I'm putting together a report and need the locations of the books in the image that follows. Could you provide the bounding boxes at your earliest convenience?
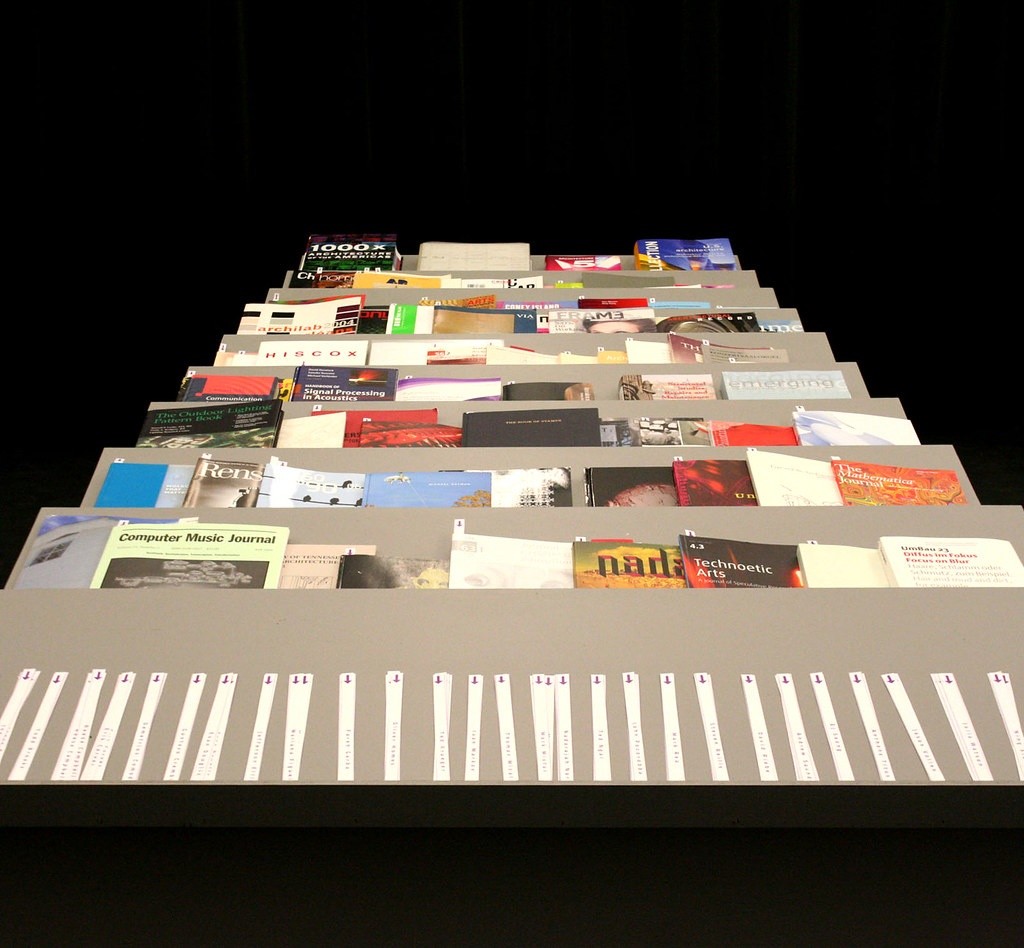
[16,231,968,593]
[879,535,1024,589]
